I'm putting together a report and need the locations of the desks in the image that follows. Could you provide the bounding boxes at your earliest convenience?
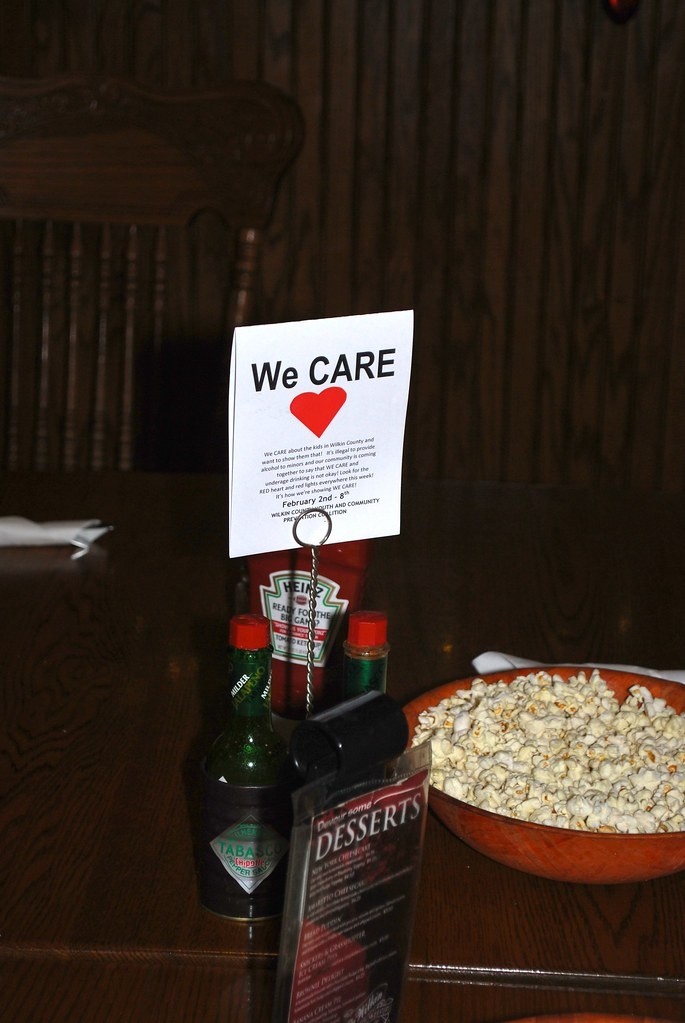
[0,471,685,1023]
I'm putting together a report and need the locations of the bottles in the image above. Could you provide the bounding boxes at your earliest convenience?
[246,534,367,719]
[340,612,390,697]
[187,614,291,920]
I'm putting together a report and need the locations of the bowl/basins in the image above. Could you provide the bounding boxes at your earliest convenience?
[398,666,684,887]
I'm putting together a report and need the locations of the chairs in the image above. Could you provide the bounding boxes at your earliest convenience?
[1,72,304,474]
